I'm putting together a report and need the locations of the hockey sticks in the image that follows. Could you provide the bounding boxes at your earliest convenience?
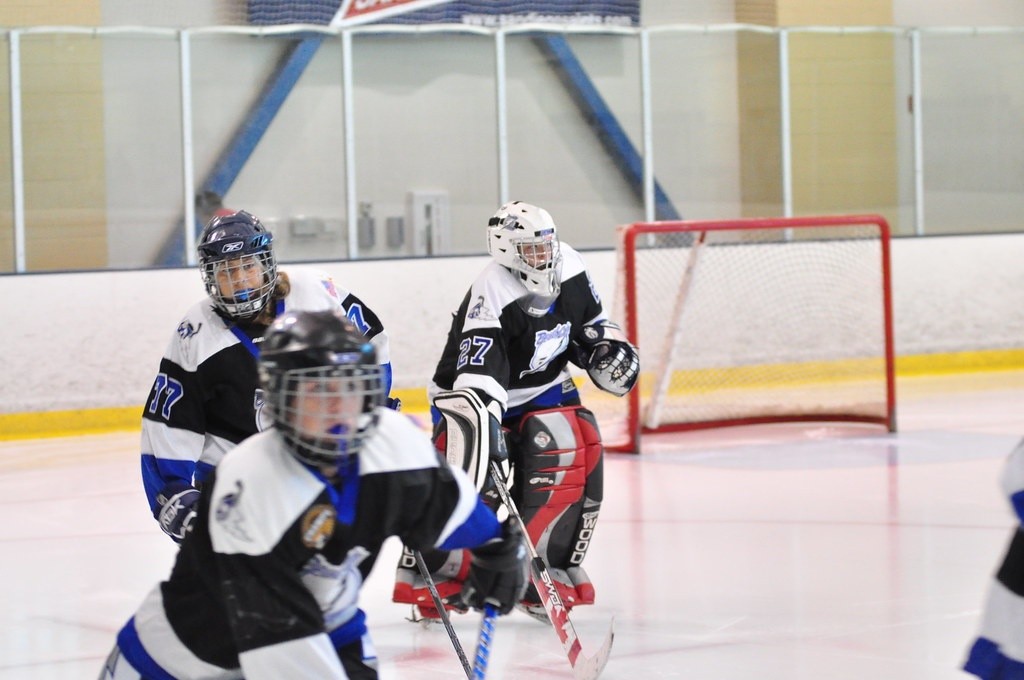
[469,598,501,680]
[488,460,617,680]
[412,550,472,678]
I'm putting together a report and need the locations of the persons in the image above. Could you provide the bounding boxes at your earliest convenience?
[96,312,500,680]
[960,435,1024,680]
[140,210,389,544]
[195,191,236,226]
[392,202,639,622]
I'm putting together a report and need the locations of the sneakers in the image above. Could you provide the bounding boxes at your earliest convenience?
[514,602,552,626]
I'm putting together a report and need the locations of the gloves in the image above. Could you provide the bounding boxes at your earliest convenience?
[159,487,201,545]
[460,523,529,615]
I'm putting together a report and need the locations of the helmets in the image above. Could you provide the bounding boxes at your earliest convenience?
[198,209,272,320]
[487,200,556,289]
[261,309,376,463]
